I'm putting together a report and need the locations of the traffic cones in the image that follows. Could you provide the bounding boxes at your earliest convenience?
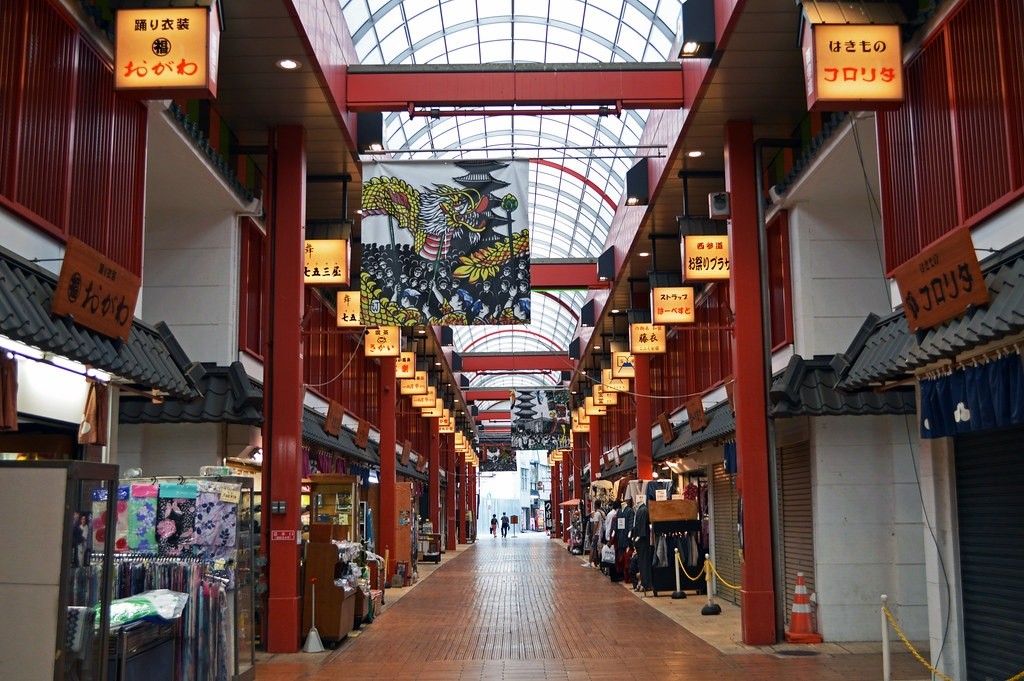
[784,572,823,644]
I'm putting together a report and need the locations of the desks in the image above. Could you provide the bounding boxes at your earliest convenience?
[93,610,184,681]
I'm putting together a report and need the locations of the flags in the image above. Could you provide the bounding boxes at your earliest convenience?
[357,157,532,326]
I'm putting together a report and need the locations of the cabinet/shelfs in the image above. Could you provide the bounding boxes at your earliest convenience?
[418,533,441,564]
[307,474,361,541]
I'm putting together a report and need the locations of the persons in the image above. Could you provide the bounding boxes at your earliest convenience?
[581,499,617,570]
[491,514,499,538]
[612,502,652,593]
[500,512,509,538]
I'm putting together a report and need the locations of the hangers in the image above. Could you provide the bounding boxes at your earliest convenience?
[215,474,222,482]
[179,475,184,484]
[91,552,204,563]
[151,476,157,484]
